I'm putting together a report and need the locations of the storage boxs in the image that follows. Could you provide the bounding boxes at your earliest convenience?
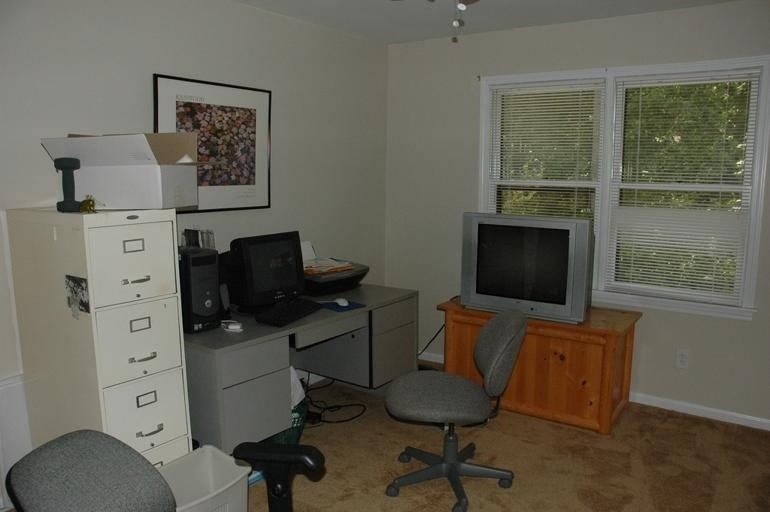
[38,132,198,210]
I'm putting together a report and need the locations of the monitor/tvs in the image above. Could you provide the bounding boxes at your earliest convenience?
[219,231,307,313]
[462,212,595,325]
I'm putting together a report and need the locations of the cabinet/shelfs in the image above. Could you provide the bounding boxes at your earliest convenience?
[436,297,642,433]
[7,208,193,469]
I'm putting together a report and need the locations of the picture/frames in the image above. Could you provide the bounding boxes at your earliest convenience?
[154,74,271,212]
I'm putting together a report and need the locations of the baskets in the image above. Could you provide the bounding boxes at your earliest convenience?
[271,410,306,445]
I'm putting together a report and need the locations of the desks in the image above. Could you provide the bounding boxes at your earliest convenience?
[184,284,419,455]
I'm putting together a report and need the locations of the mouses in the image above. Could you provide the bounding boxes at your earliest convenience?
[334,297,350,307]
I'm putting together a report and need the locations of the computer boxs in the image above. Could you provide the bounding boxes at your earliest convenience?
[178,248,220,319]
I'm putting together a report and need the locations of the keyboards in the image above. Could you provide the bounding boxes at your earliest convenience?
[255,298,323,327]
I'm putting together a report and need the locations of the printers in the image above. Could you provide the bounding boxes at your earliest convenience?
[300,240,371,297]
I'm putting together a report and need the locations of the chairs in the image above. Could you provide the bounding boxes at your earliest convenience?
[5,429,177,512]
[383,310,527,512]
